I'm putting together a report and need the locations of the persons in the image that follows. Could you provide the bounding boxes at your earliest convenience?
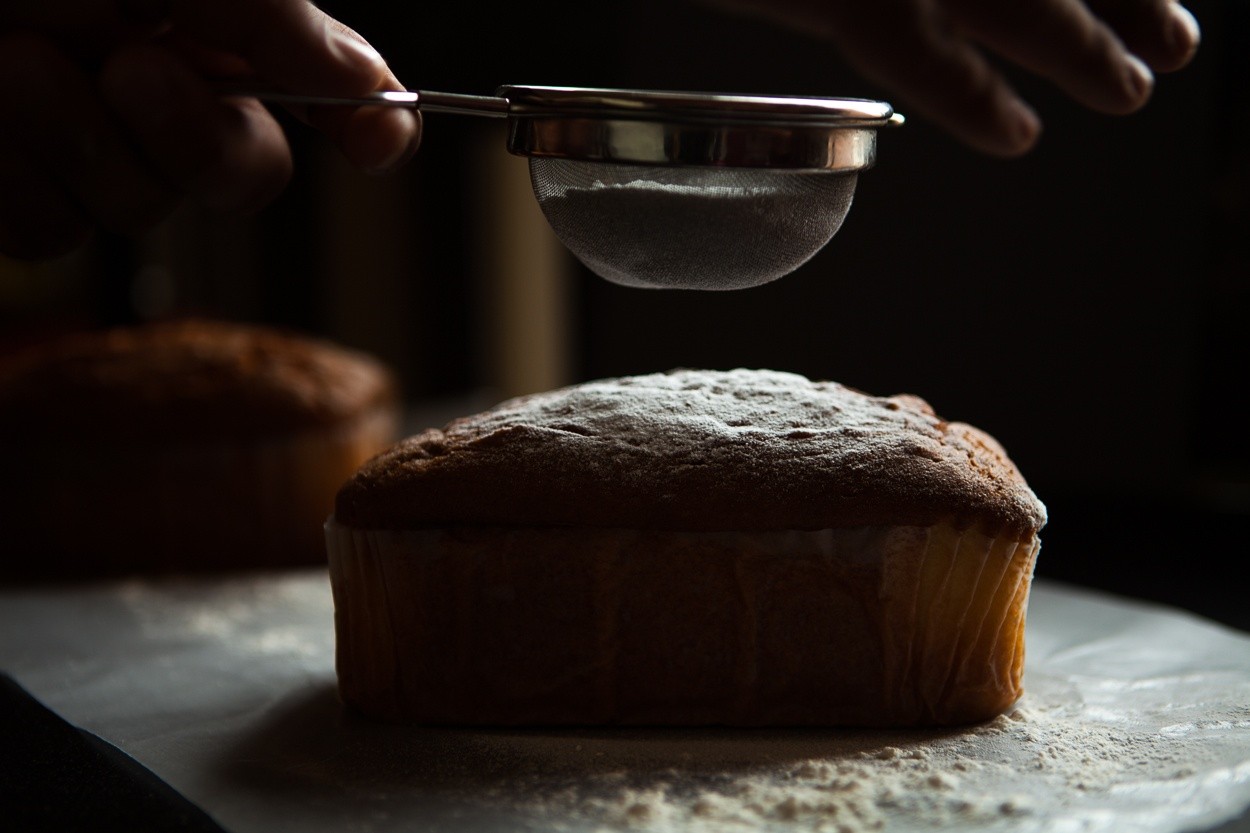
[0,0,1250,631]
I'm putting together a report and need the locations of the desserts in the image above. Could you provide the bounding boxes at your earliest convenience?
[0,325,398,578]
[323,368,1048,731]
